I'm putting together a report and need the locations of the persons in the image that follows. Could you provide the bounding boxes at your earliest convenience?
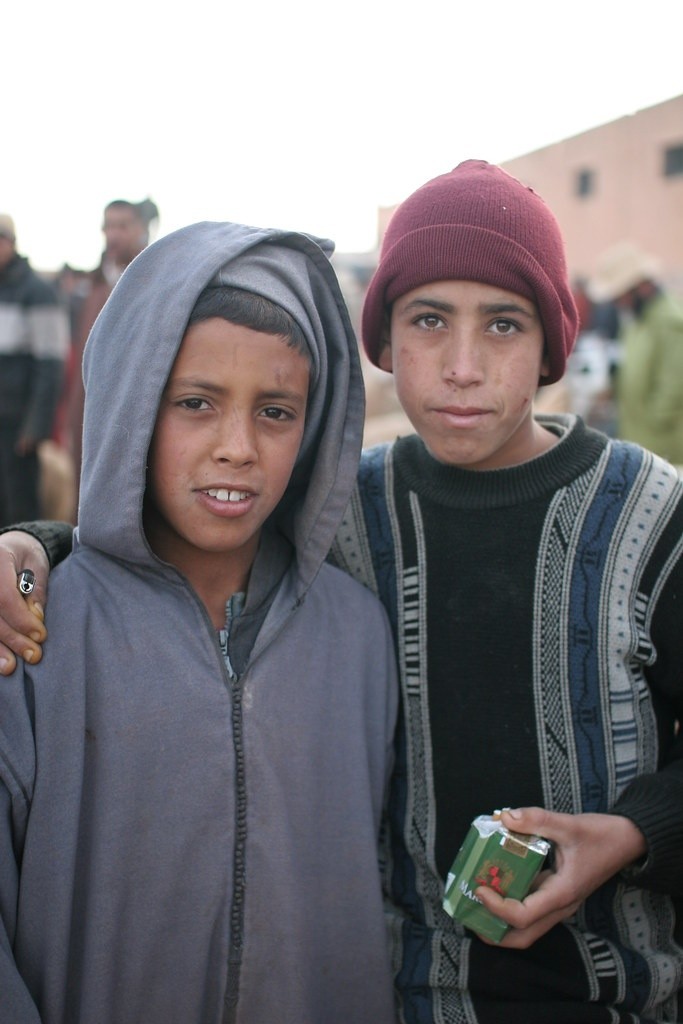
[0,222,404,1024]
[555,243,683,467]
[0,199,158,532]
[0,157,683,1024]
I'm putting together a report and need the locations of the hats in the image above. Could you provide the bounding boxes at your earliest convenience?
[361,158,580,387]
[584,240,661,303]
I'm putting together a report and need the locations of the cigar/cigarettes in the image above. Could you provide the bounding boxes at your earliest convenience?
[492,809,502,820]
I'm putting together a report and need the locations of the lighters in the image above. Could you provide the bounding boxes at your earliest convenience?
[16,568,35,598]
